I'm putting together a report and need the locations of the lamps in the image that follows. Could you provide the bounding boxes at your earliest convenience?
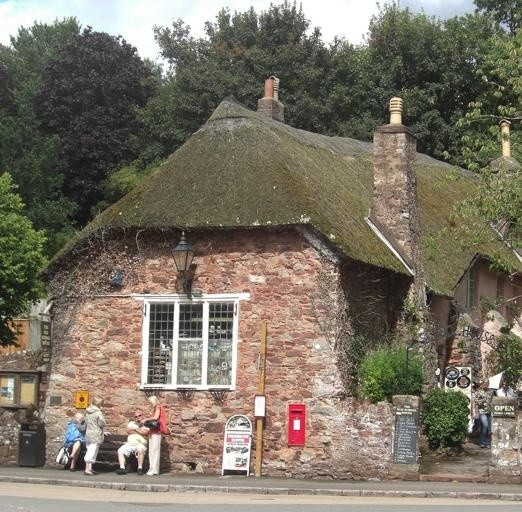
[168,232,195,286]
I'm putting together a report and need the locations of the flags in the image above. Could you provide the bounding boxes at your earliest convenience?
[488,372,502,390]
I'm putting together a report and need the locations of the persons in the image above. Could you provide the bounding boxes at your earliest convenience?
[465,377,520,450]
[115,396,167,476]
[55,396,106,476]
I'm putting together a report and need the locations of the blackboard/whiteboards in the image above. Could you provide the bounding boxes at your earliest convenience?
[392,394,420,465]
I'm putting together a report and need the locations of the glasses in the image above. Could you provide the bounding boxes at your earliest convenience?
[134,414,141,418]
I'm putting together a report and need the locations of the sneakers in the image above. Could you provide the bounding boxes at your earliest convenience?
[145,470,158,476]
[115,467,144,475]
[70,467,81,472]
[84,469,100,476]
[67,455,74,461]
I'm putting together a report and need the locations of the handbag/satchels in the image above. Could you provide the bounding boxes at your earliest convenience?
[143,418,161,428]
[54,445,72,466]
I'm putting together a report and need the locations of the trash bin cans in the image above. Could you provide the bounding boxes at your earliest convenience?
[19,417,46,467]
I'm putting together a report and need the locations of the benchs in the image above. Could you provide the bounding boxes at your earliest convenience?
[63,432,149,474]
